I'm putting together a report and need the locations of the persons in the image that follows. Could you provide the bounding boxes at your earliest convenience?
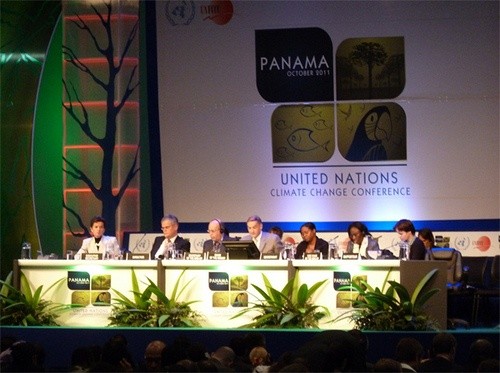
[239,215,285,259]
[150,215,191,260]
[346,222,380,259]
[393,219,425,260]
[0,329,500,373]
[203,218,239,260]
[418,229,441,251]
[73,217,123,260]
[294,223,329,259]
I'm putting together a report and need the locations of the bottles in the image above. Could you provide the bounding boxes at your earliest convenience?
[21,240,31,259]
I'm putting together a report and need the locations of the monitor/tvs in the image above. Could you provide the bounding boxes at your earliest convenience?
[222,240,260,259]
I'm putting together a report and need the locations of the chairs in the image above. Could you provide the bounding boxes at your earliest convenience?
[475,256,500,327]
[460,257,489,327]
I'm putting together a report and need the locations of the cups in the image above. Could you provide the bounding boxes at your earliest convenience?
[330,246,335,259]
[401,243,407,260]
[286,244,292,259]
[66,250,73,259]
[177,250,183,259]
[118,251,123,260]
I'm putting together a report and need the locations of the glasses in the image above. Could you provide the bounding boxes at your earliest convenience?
[160,227,170,230]
[207,229,215,233]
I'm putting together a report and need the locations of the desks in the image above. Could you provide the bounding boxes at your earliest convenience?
[13,258,447,330]
